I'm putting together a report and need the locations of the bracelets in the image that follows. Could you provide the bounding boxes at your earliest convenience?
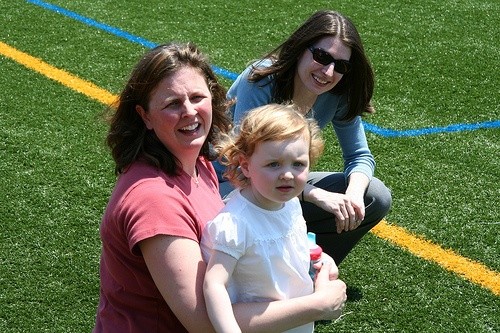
[302,190,305,202]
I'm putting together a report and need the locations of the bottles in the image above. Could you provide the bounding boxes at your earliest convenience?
[307,232,322,284]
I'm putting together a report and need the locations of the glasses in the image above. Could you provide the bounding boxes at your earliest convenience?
[308,45,352,75]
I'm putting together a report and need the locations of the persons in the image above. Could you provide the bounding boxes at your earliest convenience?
[207,11,391,299]
[96,43,348,333]
[199,103,316,333]
